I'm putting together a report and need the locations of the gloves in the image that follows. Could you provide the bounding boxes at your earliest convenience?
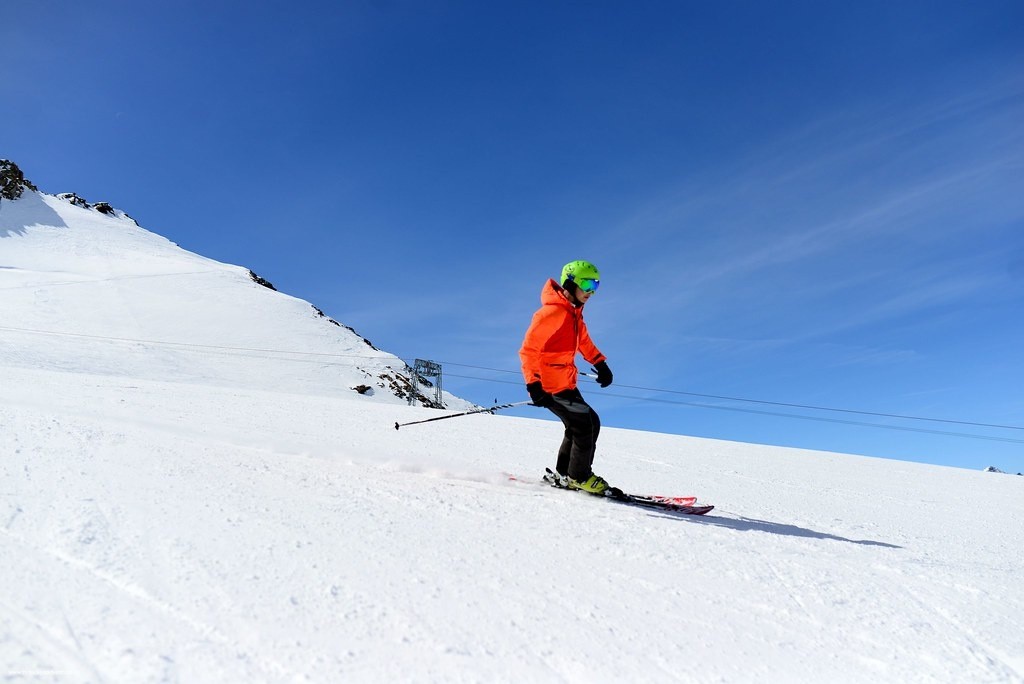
[594,361,613,388]
[526,380,552,408]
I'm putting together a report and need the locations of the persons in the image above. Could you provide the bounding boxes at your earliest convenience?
[519,261,613,493]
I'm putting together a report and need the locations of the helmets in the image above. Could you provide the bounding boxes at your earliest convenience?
[560,260,600,287]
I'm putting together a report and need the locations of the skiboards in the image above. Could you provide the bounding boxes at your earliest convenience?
[543,463,715,516]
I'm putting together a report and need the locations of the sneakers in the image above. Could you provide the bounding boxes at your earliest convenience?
[555,471,568,487]
[567,473,608,494]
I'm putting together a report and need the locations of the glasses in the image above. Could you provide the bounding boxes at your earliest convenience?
[580,279,600,292]
